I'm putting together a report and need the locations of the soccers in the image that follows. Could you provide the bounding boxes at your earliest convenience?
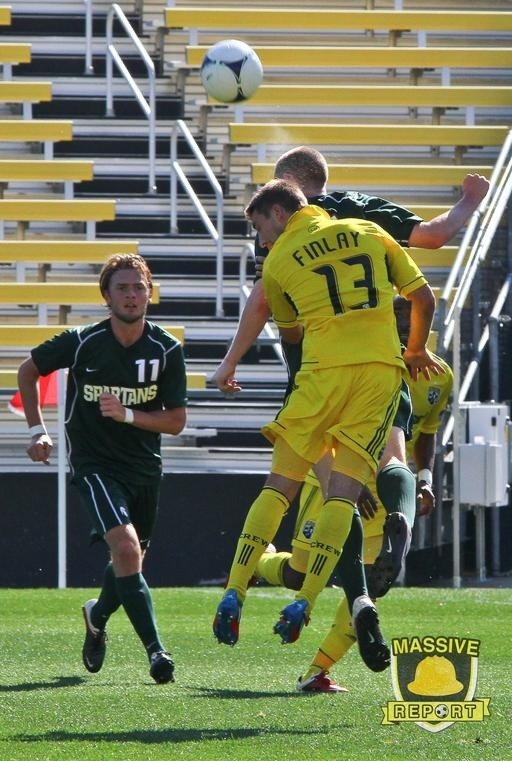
[197,38,264,105]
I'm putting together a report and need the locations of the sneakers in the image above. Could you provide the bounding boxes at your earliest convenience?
[81,597,109,674]
[210,585,244,647]
[147,650,175,686]
[223,542,277,591]
[272,599,309,646]
[297,668,348,697]
[350,596,392,675]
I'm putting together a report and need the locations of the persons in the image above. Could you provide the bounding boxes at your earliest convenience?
[210,178,444,648]
[295,292,456,697]
[19,252,188,687]
[249,146,492,678]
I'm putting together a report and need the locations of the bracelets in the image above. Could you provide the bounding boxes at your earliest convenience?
[124,407,135,425]
[28,424,48,438]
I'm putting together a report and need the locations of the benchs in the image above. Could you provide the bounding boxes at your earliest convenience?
[1,0,512,476]
